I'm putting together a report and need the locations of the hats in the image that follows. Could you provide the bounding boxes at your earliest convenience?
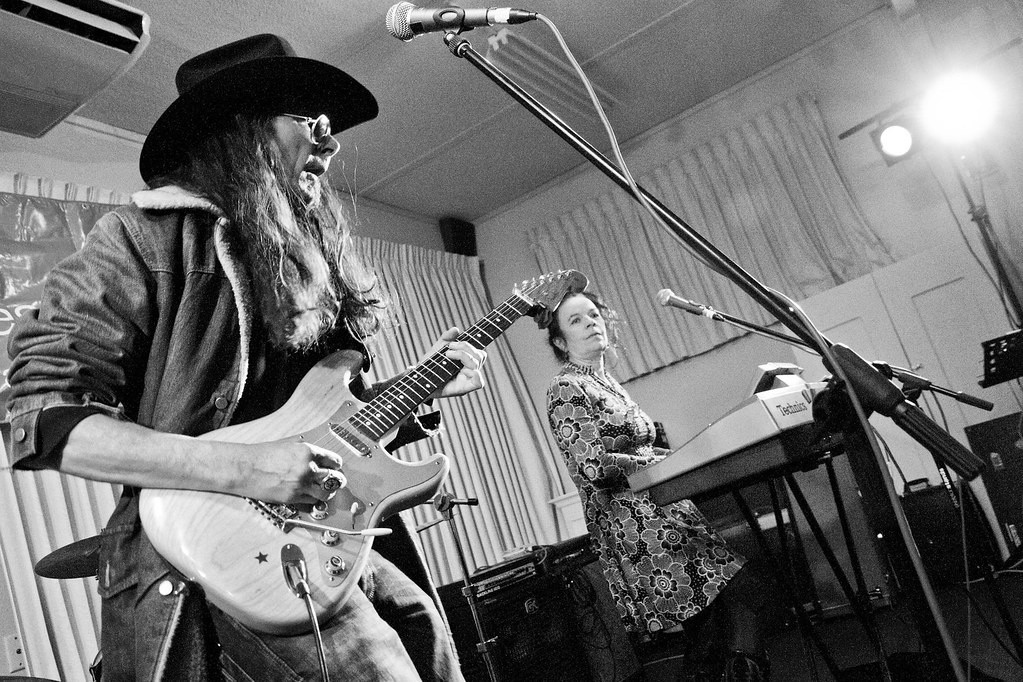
[138,32,380,190]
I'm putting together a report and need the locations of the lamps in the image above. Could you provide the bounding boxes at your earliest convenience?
[486,28,623,127]
[837,30,1022,329]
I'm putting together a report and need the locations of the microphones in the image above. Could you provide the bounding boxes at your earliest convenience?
[656,288,724,322]
[386,0,538,42]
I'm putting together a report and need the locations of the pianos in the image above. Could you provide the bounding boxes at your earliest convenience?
[624,360,862,507]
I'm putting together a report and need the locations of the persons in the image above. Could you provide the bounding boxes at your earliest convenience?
[544,290,751,682]
[0,31,488,682]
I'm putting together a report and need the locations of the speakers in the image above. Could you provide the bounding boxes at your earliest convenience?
[436,532,641,682]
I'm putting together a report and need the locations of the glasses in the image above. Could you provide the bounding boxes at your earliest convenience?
[280,112,332,145]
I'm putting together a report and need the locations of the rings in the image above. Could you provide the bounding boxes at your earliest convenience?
[318,468,342,492]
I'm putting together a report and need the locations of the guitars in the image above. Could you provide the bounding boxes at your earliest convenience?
[137,269,588,635]
